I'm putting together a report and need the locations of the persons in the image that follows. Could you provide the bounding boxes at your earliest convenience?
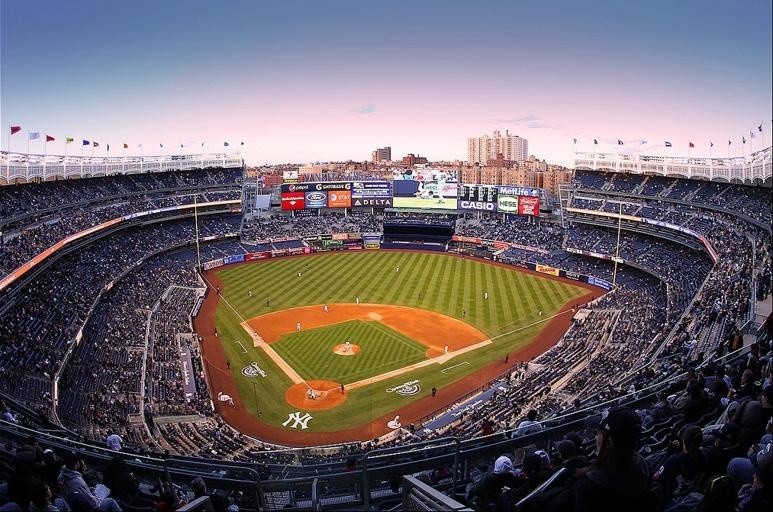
[339,383,346,394]
[307,388,320,402]
[444,345,449,356]
[266,298,274,309]
[342,341,356,351]
[295,319,303,331]
[229,400,484,512]
[321,304,329,313]
[429,386,438,398]
[460,307,467,318]
[417,291,422,300]
[356,297,359,307]
[248,287,253,297]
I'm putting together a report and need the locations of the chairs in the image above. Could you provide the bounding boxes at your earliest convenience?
[0,449,157,512]
[486,346,771,404]
[448,404,773,511]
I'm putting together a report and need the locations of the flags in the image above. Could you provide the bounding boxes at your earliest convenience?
[10,126,20,135]
[572,122,763,149]
[30,132,40,140]
[45,135,244,151]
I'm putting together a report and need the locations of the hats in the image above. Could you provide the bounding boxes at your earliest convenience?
[459,344,771,505]
